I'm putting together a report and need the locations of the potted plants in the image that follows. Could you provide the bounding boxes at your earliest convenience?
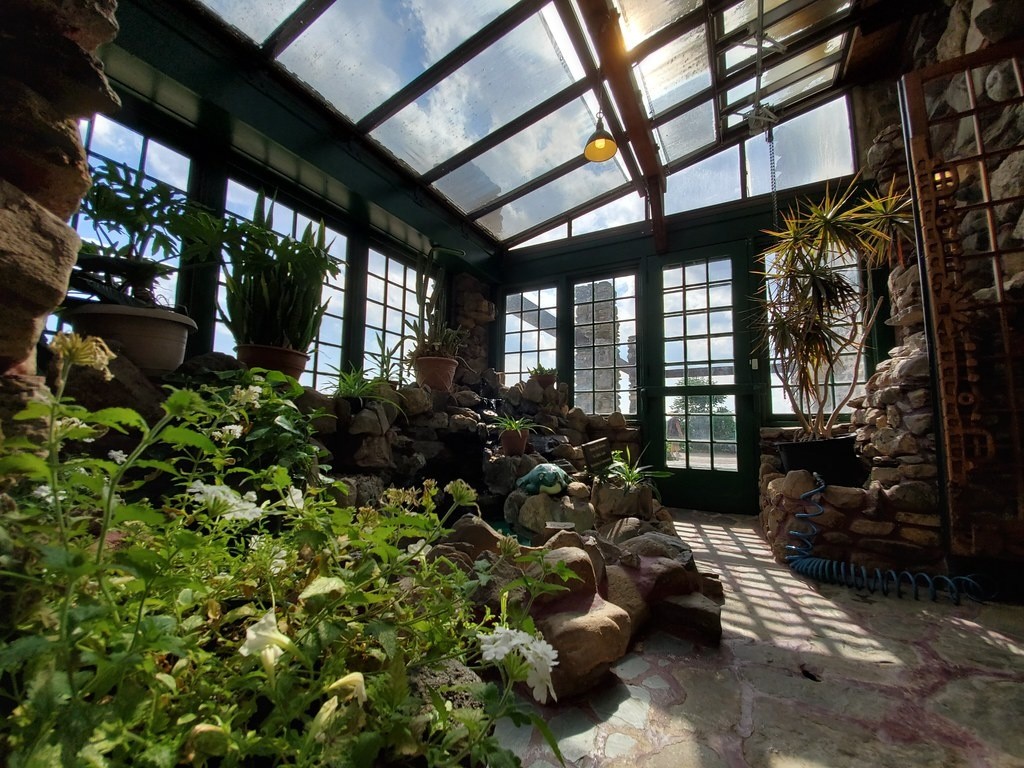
[491,412,556,457]
[50,169,349,387]
[391,247,477,391]
[324,360,409,425]
[738,168,915,488]
[360,331,416,391]
[527,362,559,388]
[589,441,675,519]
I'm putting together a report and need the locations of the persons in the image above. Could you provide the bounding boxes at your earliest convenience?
[666,410,684,457]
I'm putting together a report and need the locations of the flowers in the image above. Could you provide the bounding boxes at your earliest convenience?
[0,325,586,768]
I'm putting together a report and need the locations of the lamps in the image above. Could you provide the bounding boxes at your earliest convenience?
[583,15,618,163]
